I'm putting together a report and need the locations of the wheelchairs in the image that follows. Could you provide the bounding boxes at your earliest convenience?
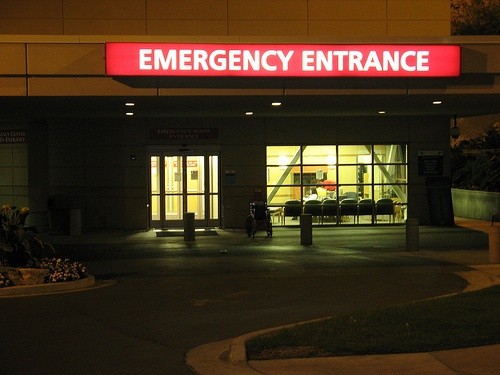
[246,199,273,238]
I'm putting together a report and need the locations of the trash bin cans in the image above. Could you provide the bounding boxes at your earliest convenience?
[47,206,71,235]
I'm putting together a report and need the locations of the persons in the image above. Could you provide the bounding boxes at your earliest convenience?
[315,185,327,197]
[320,179,336,191]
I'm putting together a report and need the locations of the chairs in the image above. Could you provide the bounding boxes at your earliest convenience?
[322,200,340,224]
[376,199,395,223]
[304,200,322,225]
[358,199,375,224]
[340,199,358,224]
[281,200,301,225]
